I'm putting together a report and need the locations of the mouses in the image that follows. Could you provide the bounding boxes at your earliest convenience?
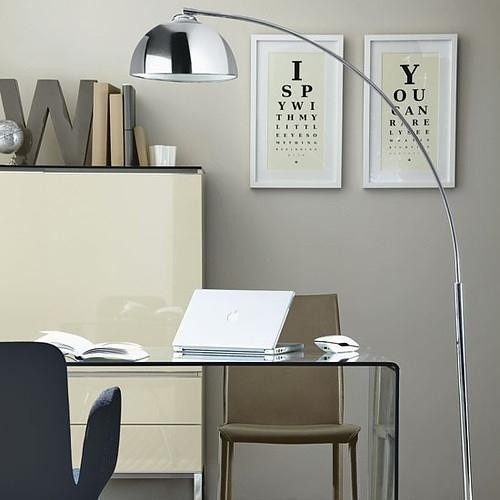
[314,336,360,352]
[316,352,360,362]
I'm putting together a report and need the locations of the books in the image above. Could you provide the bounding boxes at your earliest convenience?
[122,82,136,166]
[34,330,152,361]
[132,126,150,166]
[91,81,122,166]
[110,91,125,165]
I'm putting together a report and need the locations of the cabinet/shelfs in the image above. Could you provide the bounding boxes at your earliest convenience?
[0,164,204,500]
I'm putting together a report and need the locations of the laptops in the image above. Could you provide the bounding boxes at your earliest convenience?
[174,352,303,362]
[171,288,305,355]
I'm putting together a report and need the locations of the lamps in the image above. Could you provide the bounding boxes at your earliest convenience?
[128,7,474,500]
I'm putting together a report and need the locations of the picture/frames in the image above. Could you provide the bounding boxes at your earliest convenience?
[364,34,459,190]
[250,33,344,190]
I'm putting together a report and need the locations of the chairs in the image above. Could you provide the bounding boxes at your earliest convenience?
[216,295,362,500]
[0,339,122,500]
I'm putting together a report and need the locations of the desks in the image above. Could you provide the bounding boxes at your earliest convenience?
[65,345,403,500]
